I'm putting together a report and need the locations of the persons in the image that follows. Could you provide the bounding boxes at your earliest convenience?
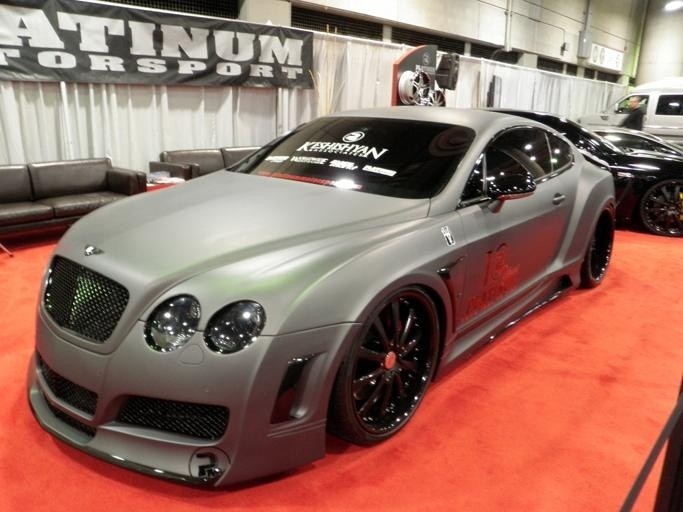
[615,94,643,131]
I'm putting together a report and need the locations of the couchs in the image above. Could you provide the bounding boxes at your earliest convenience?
[0,159,147,241]
[149,147,260,182]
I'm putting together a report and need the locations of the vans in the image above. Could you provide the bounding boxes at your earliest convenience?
[578,80,683,137]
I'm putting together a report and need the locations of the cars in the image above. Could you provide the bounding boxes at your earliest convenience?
[486,109,683,236]
[27,107,617,489]
